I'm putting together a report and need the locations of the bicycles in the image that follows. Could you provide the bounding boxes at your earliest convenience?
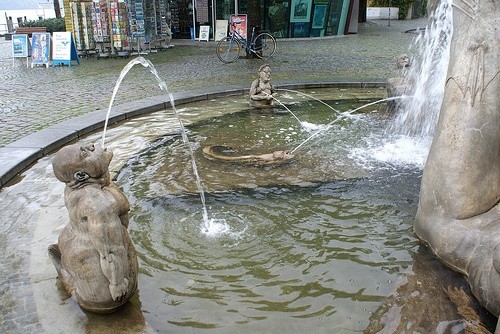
[215,21,278,64]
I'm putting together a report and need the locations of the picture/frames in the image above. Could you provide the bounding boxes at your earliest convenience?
[313,2,329,30]
[290,0,313,22]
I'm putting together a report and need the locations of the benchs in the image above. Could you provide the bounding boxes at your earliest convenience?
[16,27,46,36]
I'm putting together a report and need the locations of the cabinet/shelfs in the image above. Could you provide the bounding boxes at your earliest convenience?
[167,0,179,38]
[68,0,86,58]
[154,0,175,49]
[127,0,149,55]
[107,0,131,58]
[145,0,160,52]
[91,0,112,59]
[81,0,96,57]
